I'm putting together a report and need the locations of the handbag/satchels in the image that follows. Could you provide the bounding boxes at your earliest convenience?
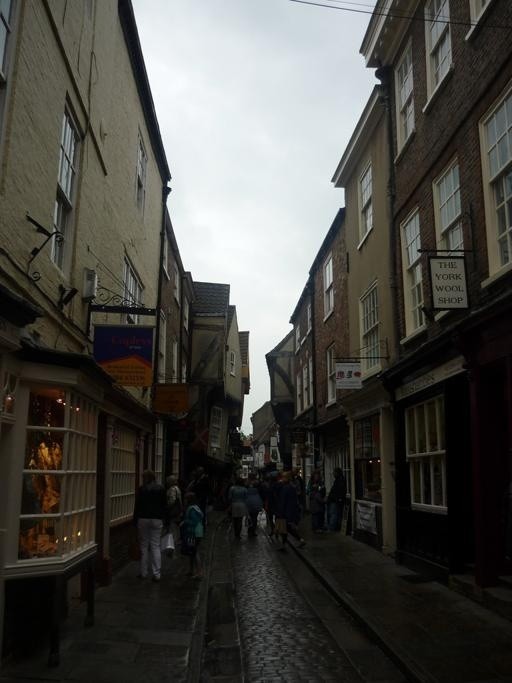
[169,498,182,518]
[257,511,267,531]
[161,533,175,551]
[182,527,195,556]
[275,519,287,534]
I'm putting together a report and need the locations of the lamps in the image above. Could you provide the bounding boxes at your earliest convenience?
[57,284,79,311]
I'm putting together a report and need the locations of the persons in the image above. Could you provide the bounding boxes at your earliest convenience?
[133,467,346,582]
